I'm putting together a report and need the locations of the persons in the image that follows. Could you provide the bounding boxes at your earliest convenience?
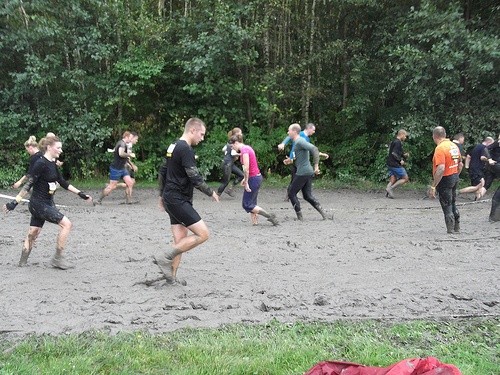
[428,126,462,232]
[228,135,278,225]
[152,118,219,281]
[456,136,496,200]
[476,139,500,199]
[97,130,139,200]
[4,133,92,270]
[277,123,329,220]
[215,128,244,196]
[450,132,466,161]
[384,129,410,198]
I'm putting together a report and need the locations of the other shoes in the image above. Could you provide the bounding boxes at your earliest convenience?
[385,188,395,199]
[223,188,236,198]
[51,256,75,270]
[93,199,101,206]
[153,251,174,282]
[267,212,279,226]
[163,278,187,287]
